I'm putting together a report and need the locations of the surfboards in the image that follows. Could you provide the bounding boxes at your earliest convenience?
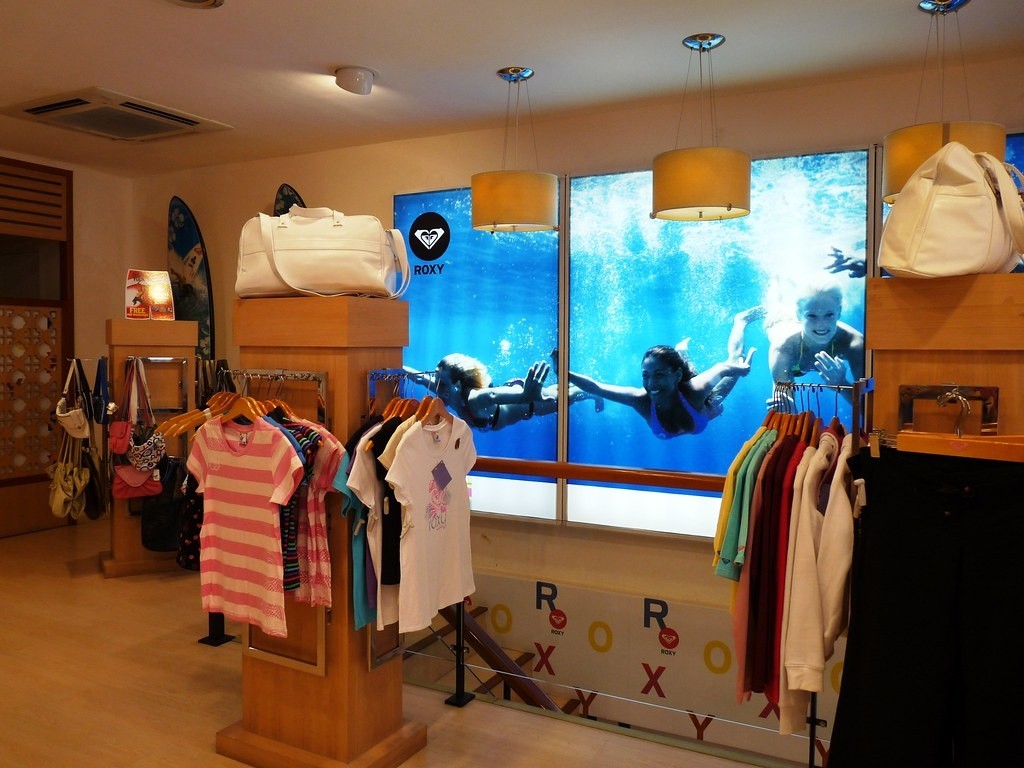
[165,193,218,362]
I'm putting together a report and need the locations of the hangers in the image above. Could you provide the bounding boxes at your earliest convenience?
[758,380,864,447]
[869,387,1024,464]
[360,369,453,432]
[153,372,295,438]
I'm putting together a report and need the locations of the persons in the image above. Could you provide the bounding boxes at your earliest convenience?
[763,273,864,390]
[554,307,765,440]
[402,349,605,442]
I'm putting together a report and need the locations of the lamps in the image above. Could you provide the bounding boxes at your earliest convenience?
[471,67,558,233]
[883,0,1005,202]
[650,33,752,220]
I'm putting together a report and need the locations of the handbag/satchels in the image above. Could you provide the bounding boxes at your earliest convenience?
[877,143,1024,276]
[48,359,188,554]
[236,206,411,298]
